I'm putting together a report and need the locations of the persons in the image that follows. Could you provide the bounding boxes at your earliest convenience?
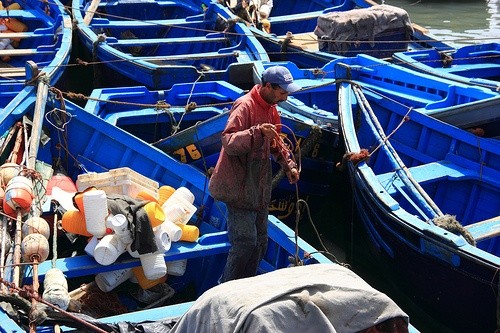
[208,66,301,285]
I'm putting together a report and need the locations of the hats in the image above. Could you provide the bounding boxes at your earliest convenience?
[261,65,302,93]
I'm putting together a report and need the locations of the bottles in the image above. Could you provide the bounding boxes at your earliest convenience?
[0,0,27,61]
[61,186,200,292]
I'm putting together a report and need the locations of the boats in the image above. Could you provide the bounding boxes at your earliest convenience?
[0,0,500,333]
